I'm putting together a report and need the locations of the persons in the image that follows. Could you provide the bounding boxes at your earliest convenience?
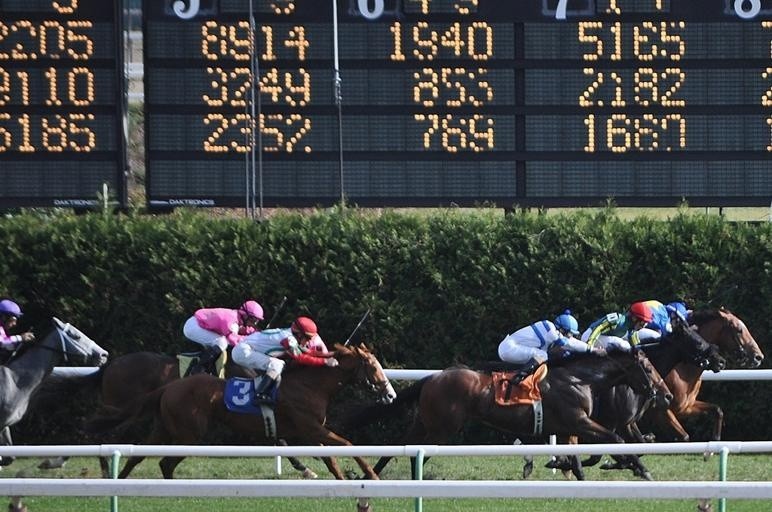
[183,299,265,364]
[627,300,687,344]
[497,313,608,390]
[0,299,35,361]
[580,302,653,361]
[231,316,338,405]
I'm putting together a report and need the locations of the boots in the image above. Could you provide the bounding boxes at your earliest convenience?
[195,345,221,375]
[255,374,276,404]
[512,357,539,385]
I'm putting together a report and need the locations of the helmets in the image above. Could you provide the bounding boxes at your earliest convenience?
[0,299,21,316]
[240,300,264,321]
[667,302,689,323]
[292,317,316,336]
[631,302,653,323]
[556,314,581,336]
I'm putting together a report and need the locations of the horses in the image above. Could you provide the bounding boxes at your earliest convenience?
[642,305,765,462]
[441,344,674,479]
[25,344,319,479]
[95,341,398,480]
[544,318,727,469]
[354,342,657,480]
[0,316,109,468]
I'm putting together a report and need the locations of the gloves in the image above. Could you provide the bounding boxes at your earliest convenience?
[595,346,607,357]
[22,332,35,341]
[326,358,338,367]
